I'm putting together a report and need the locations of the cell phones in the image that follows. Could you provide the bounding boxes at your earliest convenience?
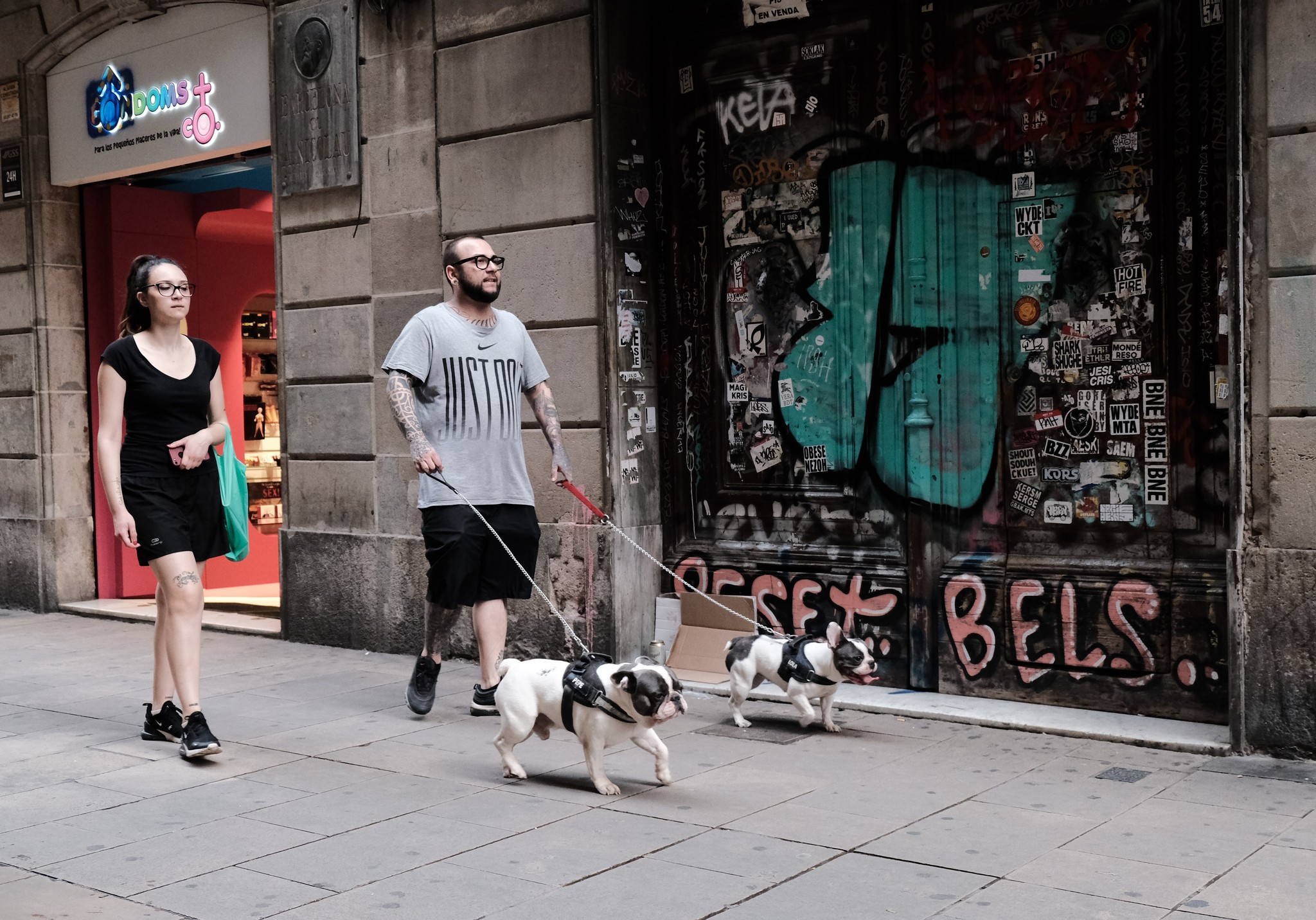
[168,447,209,467]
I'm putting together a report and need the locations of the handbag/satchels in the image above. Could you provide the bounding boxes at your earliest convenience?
[211,421,249,562]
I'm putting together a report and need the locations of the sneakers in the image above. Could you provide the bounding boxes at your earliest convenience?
[140,700,184,743]
[404,649,441,715]
[470,675,503,716]
[178,711,222,758]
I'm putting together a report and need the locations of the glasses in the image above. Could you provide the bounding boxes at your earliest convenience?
[451,255,505,270]
[142,282,196,297]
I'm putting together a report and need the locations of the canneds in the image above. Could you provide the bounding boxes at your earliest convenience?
[649,639,666,665]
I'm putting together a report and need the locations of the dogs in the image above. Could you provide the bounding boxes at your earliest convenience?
[722,621,880,734]
[493,657,688,795]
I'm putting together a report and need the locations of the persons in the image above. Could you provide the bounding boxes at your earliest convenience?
[96,254,231,761]
[380,232,574,718]
